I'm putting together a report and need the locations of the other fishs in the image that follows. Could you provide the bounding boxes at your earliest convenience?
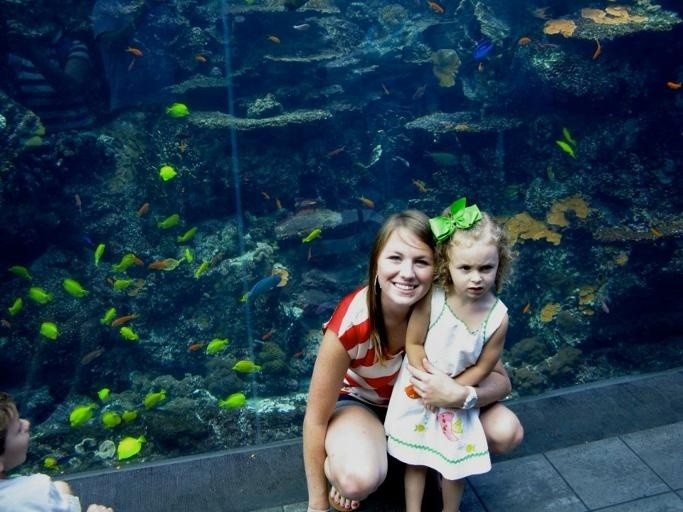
[0,191,324,473]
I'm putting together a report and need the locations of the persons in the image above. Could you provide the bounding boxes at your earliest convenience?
[0,392,113,512]
[383,196,512,511]
[301,210,524,512]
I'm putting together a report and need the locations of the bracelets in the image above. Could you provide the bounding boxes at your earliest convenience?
[308,505,329,511]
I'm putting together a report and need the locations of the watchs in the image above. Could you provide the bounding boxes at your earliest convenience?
[459,386,478,411]
